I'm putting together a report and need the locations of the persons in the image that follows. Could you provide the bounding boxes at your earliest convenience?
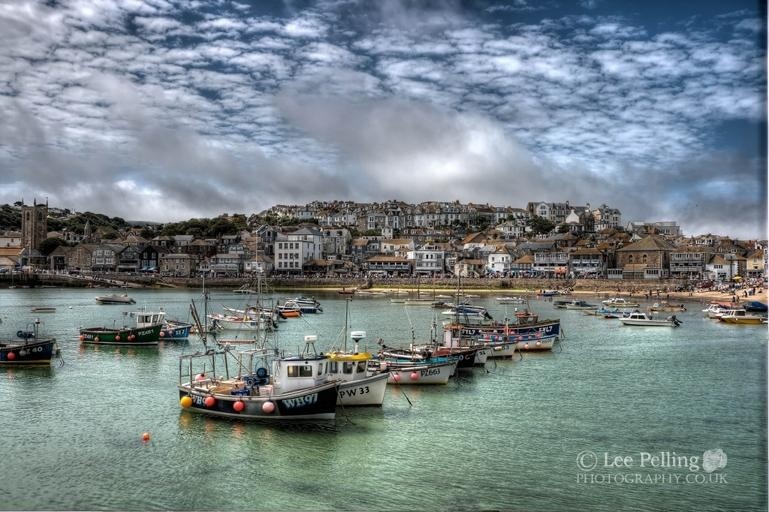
[615,274,769,304]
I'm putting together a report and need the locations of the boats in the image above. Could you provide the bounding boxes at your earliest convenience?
[119,305,191,341]
[32,307,56,314]
[0,316,56,370]
[371,264,768,384]
[95,281,137,304]
[78,322,163,346]
[176,270,344,422]
[238,263,391,410]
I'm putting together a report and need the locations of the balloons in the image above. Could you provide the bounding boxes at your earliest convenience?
[115,335,121,341]
[393,374,400,382]
[180,395,193,409]
[410,371,419,381]
[159,331,165,337]
[127,334,133,342]
[79,334,85,341]
[19,349,27,357]
[7,351,17,360]
[518,331,542,349]
[233,401,245,412]
[130,334,135,340]
[94,335,100,343]
[205,396,216,408]
[262,400,275,414]
[194,373,205,381]
[141,431,150,441]
